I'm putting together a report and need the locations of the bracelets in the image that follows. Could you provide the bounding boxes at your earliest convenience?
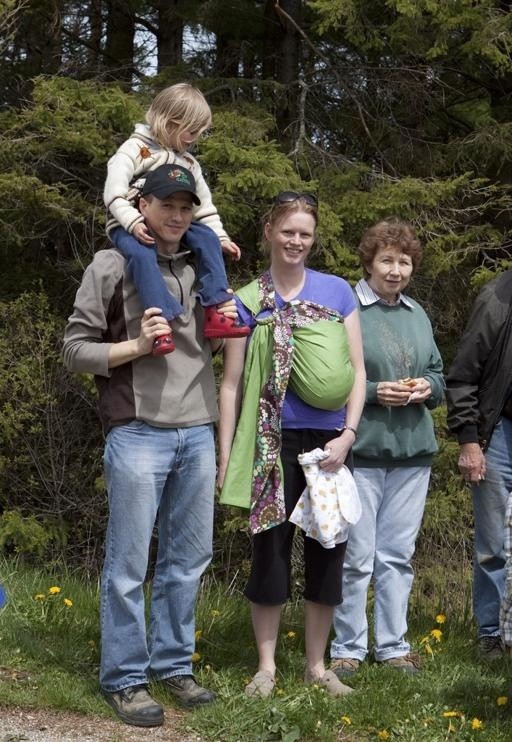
[340,426,357,437]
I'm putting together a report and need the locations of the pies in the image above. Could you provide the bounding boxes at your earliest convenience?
[399,377,416,388]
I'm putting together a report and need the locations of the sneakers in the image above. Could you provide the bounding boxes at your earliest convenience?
[202,308,250,337]
[329,658,359,677]
[476,635,505,662]
[102,683,163,727]
[245,669,276,699]
[151,324,174,355]
[384,655,419,674]
[164,675,213,709]
[305,670,353,696]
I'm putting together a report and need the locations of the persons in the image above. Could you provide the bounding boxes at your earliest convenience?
[60,166,237,726]
[215,196,366,702]
[100,83,252,355]
[329,221,446,674]
[445,272,511,662]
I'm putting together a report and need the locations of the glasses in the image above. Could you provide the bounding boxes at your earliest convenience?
[278,191,318,206]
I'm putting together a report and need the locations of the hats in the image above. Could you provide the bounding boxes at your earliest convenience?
[141,164,201,206]
